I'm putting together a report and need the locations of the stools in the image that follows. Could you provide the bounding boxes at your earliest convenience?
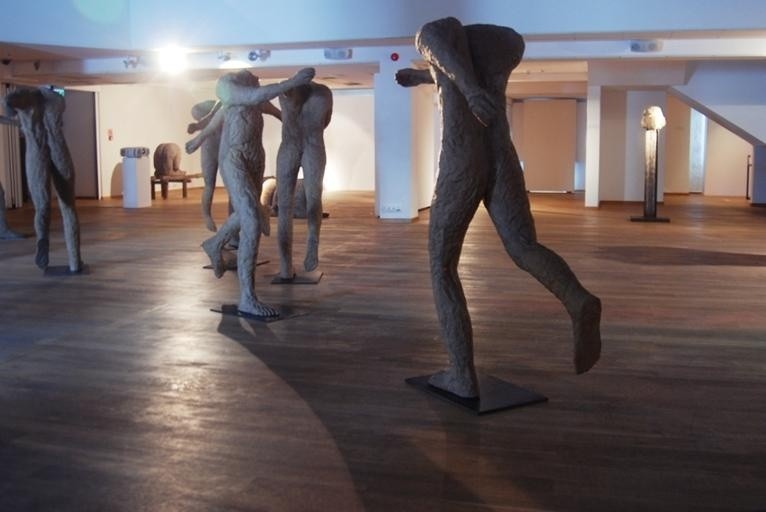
[153,177,192,200]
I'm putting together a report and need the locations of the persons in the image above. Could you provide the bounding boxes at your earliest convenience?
[393,17,602,398]
[4,86,90,274]
[276,79,332,280]
[200,70,314,318]
[0,185,24,238]
[185,99,240,247]
